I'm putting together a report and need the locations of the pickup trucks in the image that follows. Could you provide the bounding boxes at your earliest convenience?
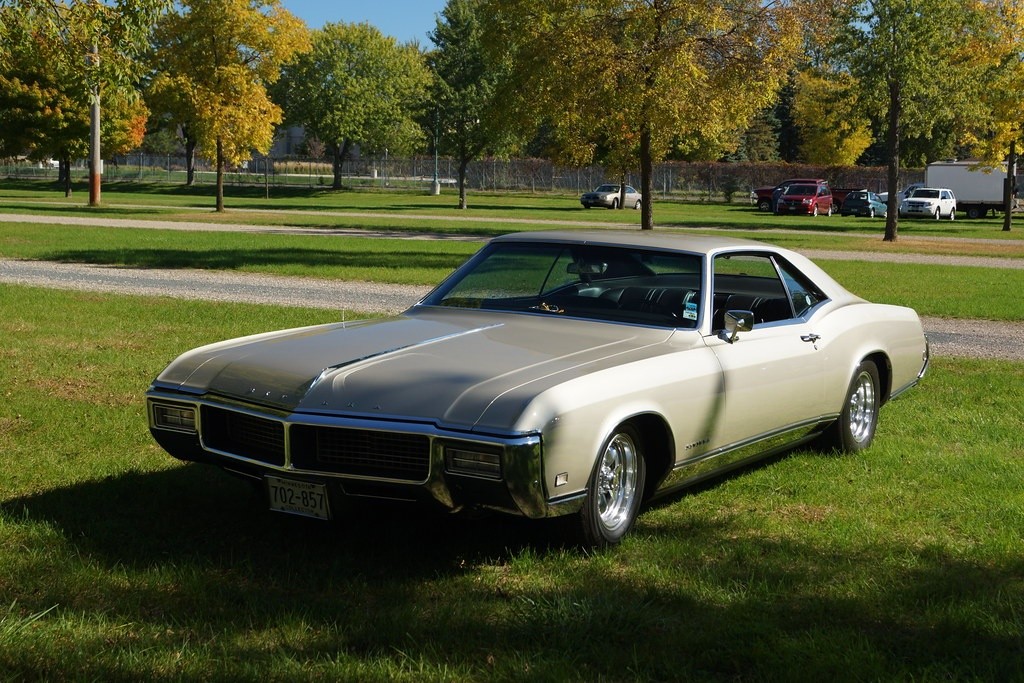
[830,187,868,213]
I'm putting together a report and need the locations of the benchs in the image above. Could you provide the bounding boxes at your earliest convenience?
[574,285,804,332]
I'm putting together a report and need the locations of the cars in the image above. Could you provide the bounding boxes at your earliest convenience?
[146,228,930,551]
[899,188,957,222]
[579,183,642,210]
[777,183,834,218]
[840,191,888,218]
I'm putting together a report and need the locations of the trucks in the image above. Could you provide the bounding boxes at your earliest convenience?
[878,160,1016,219]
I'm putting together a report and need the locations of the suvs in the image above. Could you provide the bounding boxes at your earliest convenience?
[749,178,829,211]
